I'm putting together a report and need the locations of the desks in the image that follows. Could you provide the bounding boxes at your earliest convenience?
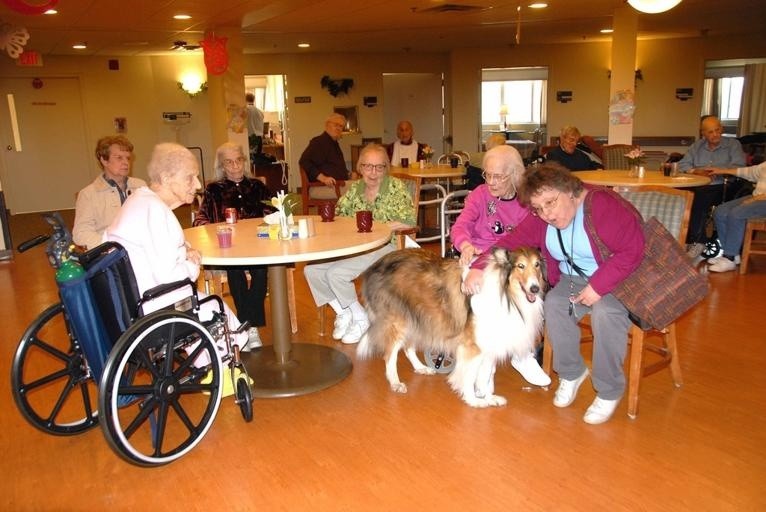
[385,164,467,245]
[184,215,392,399]
[569,169,713,189]
[505,140,536,159]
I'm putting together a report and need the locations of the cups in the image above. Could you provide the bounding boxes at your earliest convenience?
[320,204,335,222]
[356,211,373,232]
[664,162,678,177]
[216,228,232,249]
[451,158,458,168]
[401,158,408,168]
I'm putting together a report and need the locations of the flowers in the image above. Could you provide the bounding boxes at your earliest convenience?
[260,190,300,216]
[422,144,435,157]
[623,148,649,165]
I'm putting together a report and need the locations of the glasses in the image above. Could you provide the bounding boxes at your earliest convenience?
[532,192,561,216]
[482,170,508,181]
[221,159,243,163]
[563,141,582,144]
[361,164,385,171]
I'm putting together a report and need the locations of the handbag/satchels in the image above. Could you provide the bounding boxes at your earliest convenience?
[611,217,708,330]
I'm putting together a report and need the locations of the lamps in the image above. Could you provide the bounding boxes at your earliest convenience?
[626,0,683,14]
[177,81,208,98]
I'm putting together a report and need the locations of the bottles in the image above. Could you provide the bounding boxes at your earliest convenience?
[420,160,426,170]
[298,219,315,238]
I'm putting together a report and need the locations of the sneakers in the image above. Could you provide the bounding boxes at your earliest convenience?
[332,308,370,344]
[553,368,588,408]
[241,327,263,352]
[200,367,253,398]
[511,352,551,386]
[687,242,705,257]
[708,254,741,272]
[583,396,621,424]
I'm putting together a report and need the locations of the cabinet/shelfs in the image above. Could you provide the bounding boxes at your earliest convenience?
[351,143,391,175]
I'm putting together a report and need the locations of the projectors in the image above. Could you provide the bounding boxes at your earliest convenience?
[162,112,190,121]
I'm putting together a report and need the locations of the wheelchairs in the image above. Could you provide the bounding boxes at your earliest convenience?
[12,214,253,467]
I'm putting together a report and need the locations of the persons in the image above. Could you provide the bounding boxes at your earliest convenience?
[450,147,534,265]
[386,120,427,234]
[302,144,417,344]
[102,143,254,400]
[704,161,766,272]
[245,93,264,153]
[462,162,644,426]
[299,113,360,199]
[458,137,506,204]
[470,133,506,170]
[73,135,147,251]
[677,115,746,258]
[192,142,277,354]
[542,125,605,171]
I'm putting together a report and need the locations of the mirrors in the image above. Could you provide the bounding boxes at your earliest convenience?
[334,105,361,137]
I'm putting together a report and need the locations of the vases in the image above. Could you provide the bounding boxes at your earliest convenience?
[426,156,433,168]
[628,166,639,178]
[278,214,292,240]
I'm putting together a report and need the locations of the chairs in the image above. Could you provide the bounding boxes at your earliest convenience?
[299,166,345,216]
[191,176,297,335]
[543,185,695,419]
[601,144,640,170]
[540,146,556,156]
[319,173,422,337]
[739,193,766,274]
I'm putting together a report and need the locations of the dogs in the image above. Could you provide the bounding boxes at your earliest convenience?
[353,246,550,408]
